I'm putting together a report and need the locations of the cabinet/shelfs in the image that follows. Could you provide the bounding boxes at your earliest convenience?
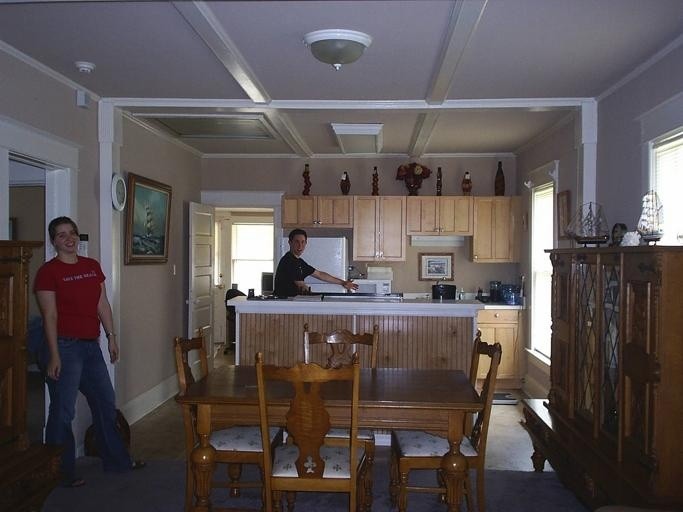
[1,239,58,512]
[351,196,407,262]
[406,196,474,235]
[477,307,524,389]
[280,195,352,228]
[471,198,523,265]
[518,246,680,511]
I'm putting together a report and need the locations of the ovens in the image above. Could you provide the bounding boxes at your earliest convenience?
[350,276,392,296]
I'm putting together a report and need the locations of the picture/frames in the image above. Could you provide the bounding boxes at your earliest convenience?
[417,252,454,283]
[125,171,170,266]
[555,190,574,242]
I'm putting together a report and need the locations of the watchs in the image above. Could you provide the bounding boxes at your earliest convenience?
[105,333,116,338]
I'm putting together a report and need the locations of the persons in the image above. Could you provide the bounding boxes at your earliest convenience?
[270,228,358,299]
[32,216,146,488]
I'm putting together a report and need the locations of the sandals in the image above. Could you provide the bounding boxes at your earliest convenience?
[123,461,146,471]
[59,478,86,487]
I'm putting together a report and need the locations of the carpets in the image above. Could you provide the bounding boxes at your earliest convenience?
[44,455,587,511]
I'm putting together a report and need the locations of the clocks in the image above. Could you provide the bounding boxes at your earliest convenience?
[111,172,127,212]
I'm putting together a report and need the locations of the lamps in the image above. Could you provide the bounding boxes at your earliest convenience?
[302,30,373,70]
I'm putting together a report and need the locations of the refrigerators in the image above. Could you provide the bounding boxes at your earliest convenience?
[280,236,349,296]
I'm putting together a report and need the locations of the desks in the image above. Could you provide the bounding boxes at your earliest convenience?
[175,366,482,512]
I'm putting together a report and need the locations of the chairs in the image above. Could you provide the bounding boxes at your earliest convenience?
[289,325,380,509]
[390,329,502,511]
[254,349,366,511]
[172,326,281,510]
